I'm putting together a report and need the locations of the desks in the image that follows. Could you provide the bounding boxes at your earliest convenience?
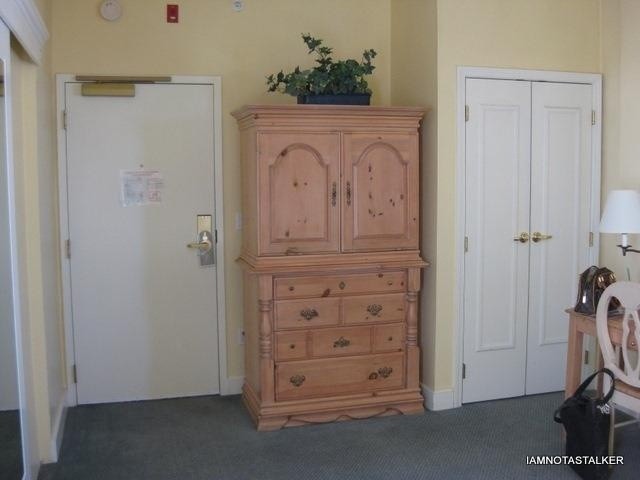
[565,308,640,400]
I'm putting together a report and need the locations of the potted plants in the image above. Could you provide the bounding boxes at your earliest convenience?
[266,33,376,105]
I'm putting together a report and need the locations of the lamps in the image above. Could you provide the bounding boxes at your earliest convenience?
[599,190,640,257]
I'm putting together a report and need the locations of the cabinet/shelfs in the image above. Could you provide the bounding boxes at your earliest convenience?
[229,104,430,272]
[241,268,425,431]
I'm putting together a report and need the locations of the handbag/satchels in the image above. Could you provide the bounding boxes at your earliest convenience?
[557,395,610,480]
[575,266,619,315]
